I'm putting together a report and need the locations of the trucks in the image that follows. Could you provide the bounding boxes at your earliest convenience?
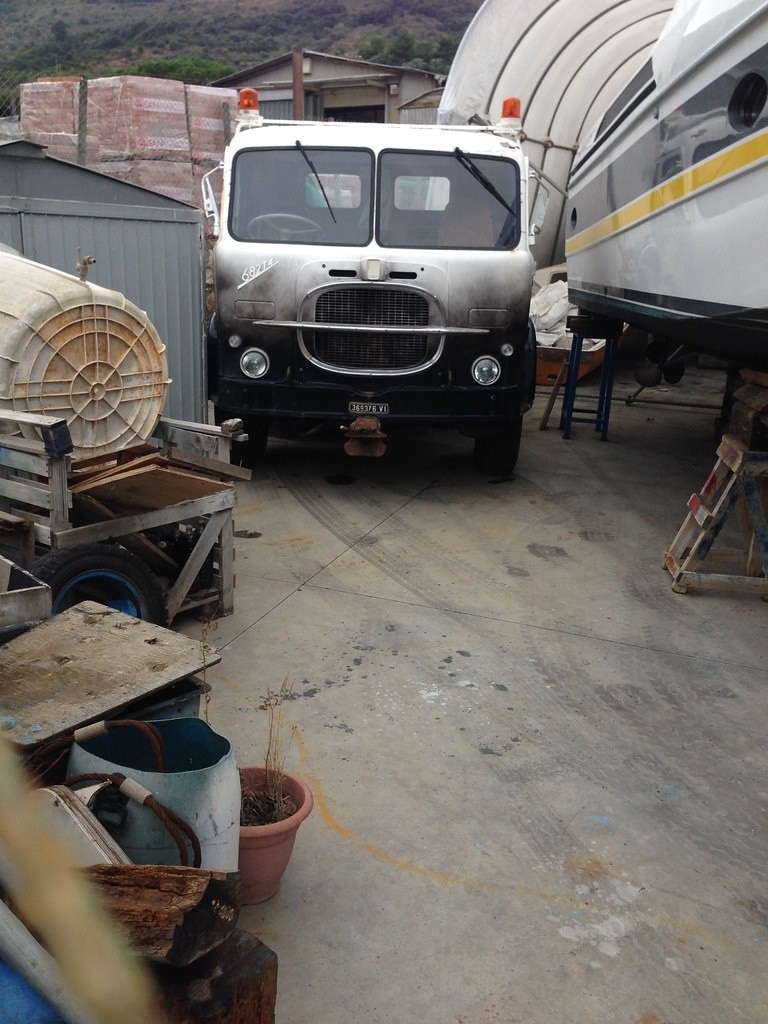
[195,87,538,486]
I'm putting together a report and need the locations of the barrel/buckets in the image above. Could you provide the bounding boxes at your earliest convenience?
[53,569,149,622]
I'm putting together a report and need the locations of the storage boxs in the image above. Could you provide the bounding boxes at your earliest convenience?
[19,74,241,235]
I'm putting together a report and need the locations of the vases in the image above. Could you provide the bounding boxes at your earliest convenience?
[215,765,315,906]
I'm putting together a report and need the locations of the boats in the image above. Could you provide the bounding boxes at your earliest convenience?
[433,2,768,330]
